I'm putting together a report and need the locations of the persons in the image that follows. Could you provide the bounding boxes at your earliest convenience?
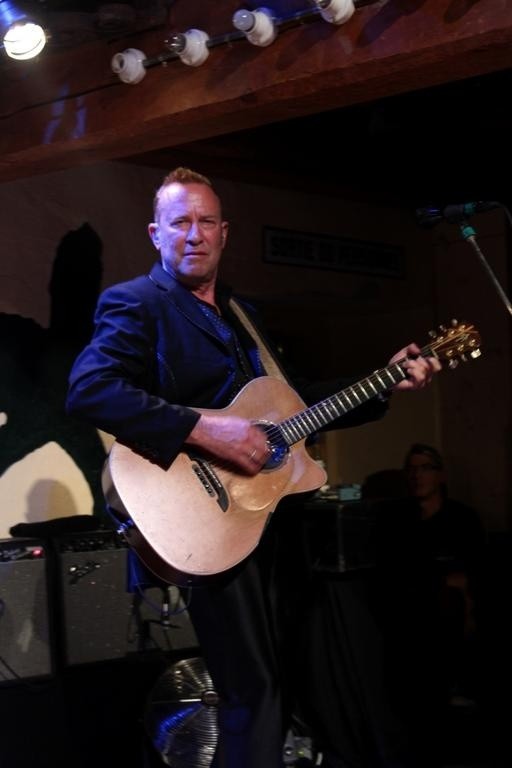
[67,165,446,763]
[373,442,485,738]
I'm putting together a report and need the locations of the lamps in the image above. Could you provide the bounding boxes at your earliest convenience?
[0,2,47,62]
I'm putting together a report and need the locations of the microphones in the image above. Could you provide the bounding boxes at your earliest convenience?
[413,199,499,231]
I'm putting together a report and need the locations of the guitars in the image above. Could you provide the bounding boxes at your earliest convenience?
[100,319,482,586]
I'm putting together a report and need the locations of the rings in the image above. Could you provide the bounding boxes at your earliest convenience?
[248,447,257,461]
